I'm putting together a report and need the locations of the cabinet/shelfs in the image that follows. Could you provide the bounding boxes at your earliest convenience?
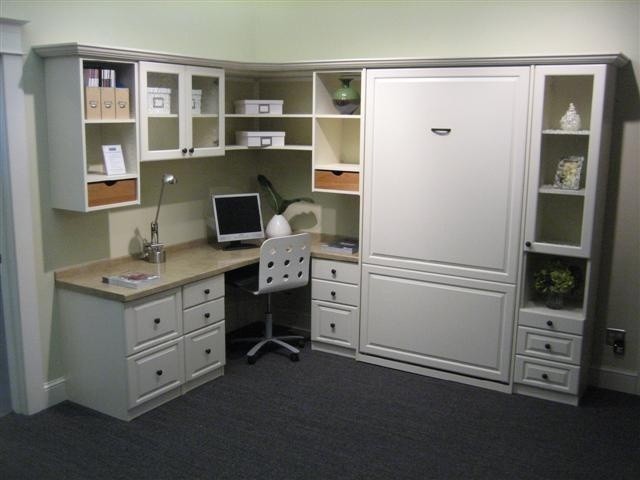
[137,61,225,165]
[121,286,185,423]
[227,64,313,149]
[43,58,140,212]
[303,64,609,407]
[181,273,226,395]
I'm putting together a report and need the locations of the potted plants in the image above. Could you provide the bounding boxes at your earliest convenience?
[533,264,583,311]
[256,177,314,238]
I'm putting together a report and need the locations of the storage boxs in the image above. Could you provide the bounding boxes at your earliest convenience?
[146,87,171,114]
[234,99,283,114]
[192,89,203,117]
[236,130,285,147]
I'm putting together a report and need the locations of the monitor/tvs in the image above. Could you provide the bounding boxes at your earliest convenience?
[211,193,265,251]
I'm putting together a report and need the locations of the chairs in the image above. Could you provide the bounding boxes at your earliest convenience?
[233,231,312,365]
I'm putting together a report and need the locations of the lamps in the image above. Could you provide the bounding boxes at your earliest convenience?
[141,173,179,263]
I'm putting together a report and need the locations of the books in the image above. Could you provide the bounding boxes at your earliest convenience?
[320,238,358,254]
[102,270,160,289]
[85,68,116,87]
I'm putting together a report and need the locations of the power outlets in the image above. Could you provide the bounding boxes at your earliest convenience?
[604,328,625,355]
